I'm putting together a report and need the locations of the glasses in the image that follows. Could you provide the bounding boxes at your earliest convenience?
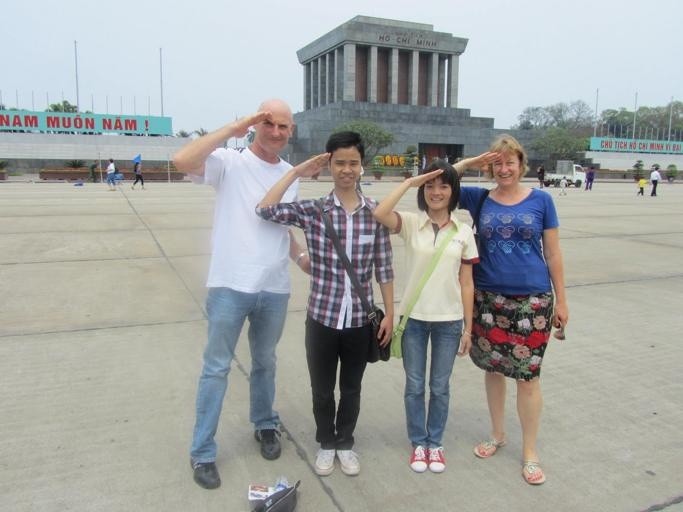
[551,316,566,340]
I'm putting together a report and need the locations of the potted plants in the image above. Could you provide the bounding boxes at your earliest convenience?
[369,154,385,180]
[400,145,420,180]
[63,160,88,171]
[664,164,680,182]
[632,160,646,182]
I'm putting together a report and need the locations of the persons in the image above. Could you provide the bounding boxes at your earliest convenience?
[253,131,394,480]
[649,166,663,197]
[636,176,648,197]
[558,176,569,196]
[89,158,145,192]
[354,165,365,193]
[372,160,480,405]
[451,134,570,486]
[536,164,546,188]
[172,98,314,489]
[583,167,595,191]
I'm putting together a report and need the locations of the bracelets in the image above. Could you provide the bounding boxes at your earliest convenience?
[294,250,308,266]
[458,161,465,178]
[462,329,475,337]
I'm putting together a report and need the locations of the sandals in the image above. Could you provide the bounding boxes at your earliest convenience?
[522,460,546,485]
[474,438,506,458]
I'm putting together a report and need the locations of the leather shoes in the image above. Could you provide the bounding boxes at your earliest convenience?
[255,430,281,459]
[191,457,220,489]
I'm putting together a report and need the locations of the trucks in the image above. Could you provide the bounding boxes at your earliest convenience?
[543,160,586,188]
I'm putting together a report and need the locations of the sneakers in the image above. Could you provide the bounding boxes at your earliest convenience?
[336,449,361,476]
[410,445,428,472]
[314,448,336,476]
[428,447,446,473]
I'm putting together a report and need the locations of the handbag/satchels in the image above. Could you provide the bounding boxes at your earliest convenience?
[367,305,392,363]
[390,324,404,359]
